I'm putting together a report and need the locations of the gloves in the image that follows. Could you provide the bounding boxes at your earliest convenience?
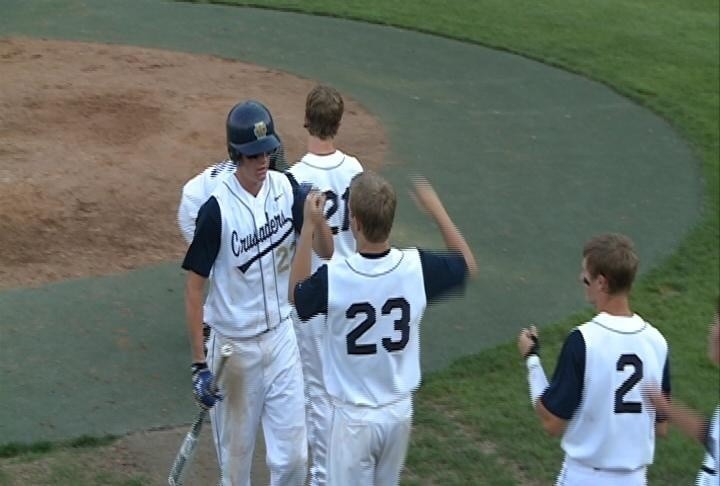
[187,360,224,411]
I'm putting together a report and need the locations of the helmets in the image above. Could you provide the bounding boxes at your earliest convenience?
[225,98,281,157]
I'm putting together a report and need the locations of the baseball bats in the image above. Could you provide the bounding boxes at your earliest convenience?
[168,342,233,485]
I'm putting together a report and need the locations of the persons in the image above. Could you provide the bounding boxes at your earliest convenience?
[177,99,336,486]
[286,173,478,485]
[283,82,365,485]
[518,233,672,486]
[179,158,240,248]
[640,294,720,486]
